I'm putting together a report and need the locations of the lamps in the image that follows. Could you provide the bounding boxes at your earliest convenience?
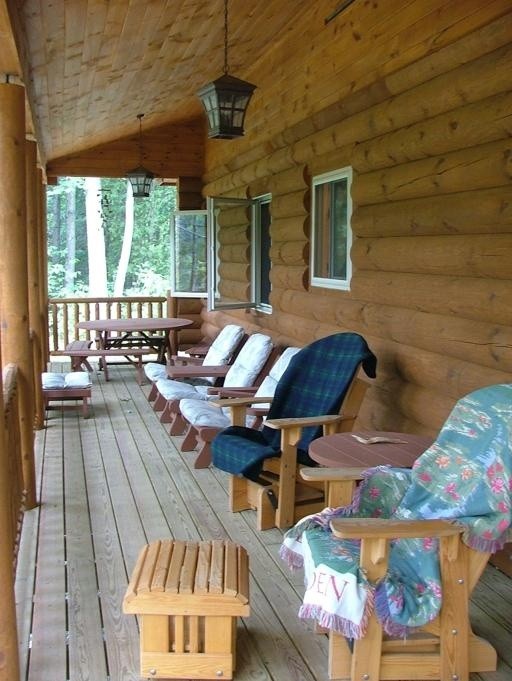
[195,1,257,140]
[125,114,155,197]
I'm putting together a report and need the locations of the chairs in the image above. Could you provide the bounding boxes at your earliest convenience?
[178,347,303,469]
[211,332,369,532]
[299,385,512,681]
[143,325,250,412]
[155,332,278,435]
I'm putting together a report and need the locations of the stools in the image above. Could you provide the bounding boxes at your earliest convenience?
[122,541,252,680]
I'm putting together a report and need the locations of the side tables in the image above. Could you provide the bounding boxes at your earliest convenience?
[307,431,435,508]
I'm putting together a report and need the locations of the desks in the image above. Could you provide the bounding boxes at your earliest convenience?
[75,318,194,385]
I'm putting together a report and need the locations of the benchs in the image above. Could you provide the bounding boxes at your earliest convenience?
[65,349,156,386]
[65,339,93,372]
[95,335,166,350]
[40,371,92,419]
